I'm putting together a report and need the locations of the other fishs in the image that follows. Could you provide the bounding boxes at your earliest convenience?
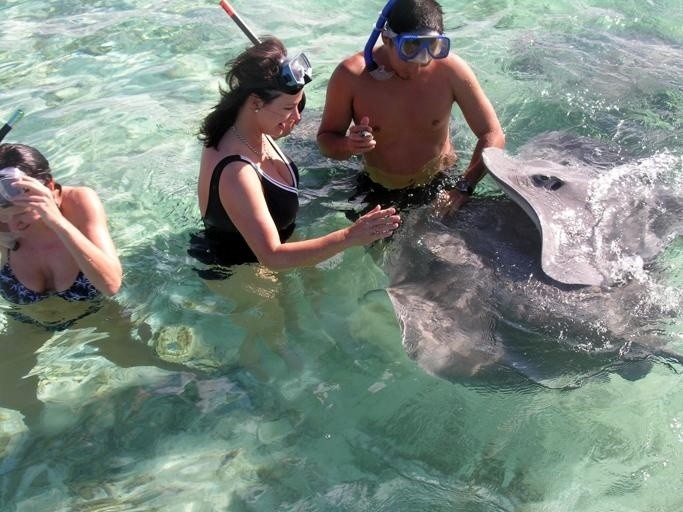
[478,118,683,287]
[362,193,683,398]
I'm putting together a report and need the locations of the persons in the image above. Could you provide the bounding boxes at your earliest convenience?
[315,0,506,277]
[184,32,405,381]
[0,138,162,428]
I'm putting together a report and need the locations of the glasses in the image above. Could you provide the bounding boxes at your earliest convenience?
[0,168,25,204]
[387,32,450,63]
[282,49,313,89]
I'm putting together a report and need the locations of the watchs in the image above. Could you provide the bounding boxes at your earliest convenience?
[453,181,474,196]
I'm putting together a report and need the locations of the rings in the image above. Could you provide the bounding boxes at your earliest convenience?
[372,227,376,233]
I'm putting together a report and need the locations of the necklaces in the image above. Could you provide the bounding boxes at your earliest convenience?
[230,125,274,166]
